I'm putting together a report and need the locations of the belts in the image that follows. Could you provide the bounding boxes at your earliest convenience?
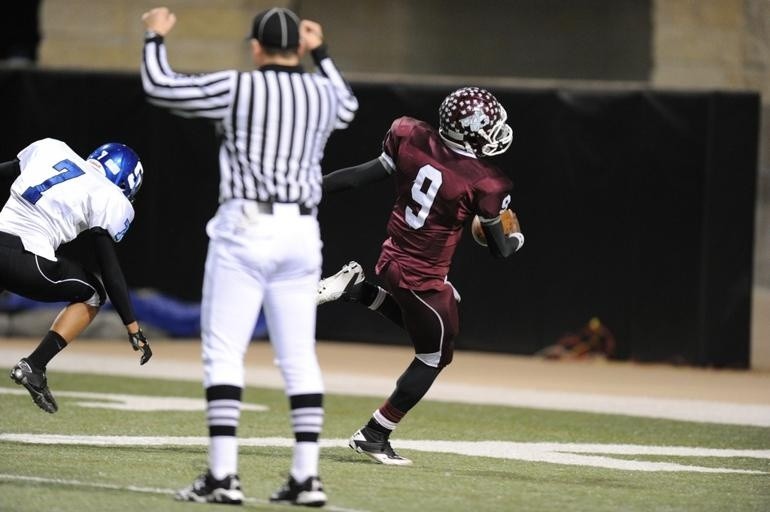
[241,203,313,215]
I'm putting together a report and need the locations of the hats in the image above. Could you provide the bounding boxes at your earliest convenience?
[243,6,302,50]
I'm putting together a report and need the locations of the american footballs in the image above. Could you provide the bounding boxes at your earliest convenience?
[472,210,513,247]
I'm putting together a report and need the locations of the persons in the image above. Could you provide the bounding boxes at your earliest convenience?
[140,7,359,505]
[0,138,152,414]
[317,87,524,468]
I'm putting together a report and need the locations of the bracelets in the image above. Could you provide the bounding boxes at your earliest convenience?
[142,33,163,44]
[509,232,524,252]
[311,45,328,65]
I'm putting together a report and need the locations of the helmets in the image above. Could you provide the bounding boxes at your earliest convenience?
[86,143,144,204]
[438,87,513,159]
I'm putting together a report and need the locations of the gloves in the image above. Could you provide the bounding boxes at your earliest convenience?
[128,328,153,366]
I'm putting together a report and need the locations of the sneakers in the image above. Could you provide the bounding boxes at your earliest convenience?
[9,357,57,413]
[174,465,244,506]
[314,260,366,306]
[348,427,414,467]
[270,472,327,506]
